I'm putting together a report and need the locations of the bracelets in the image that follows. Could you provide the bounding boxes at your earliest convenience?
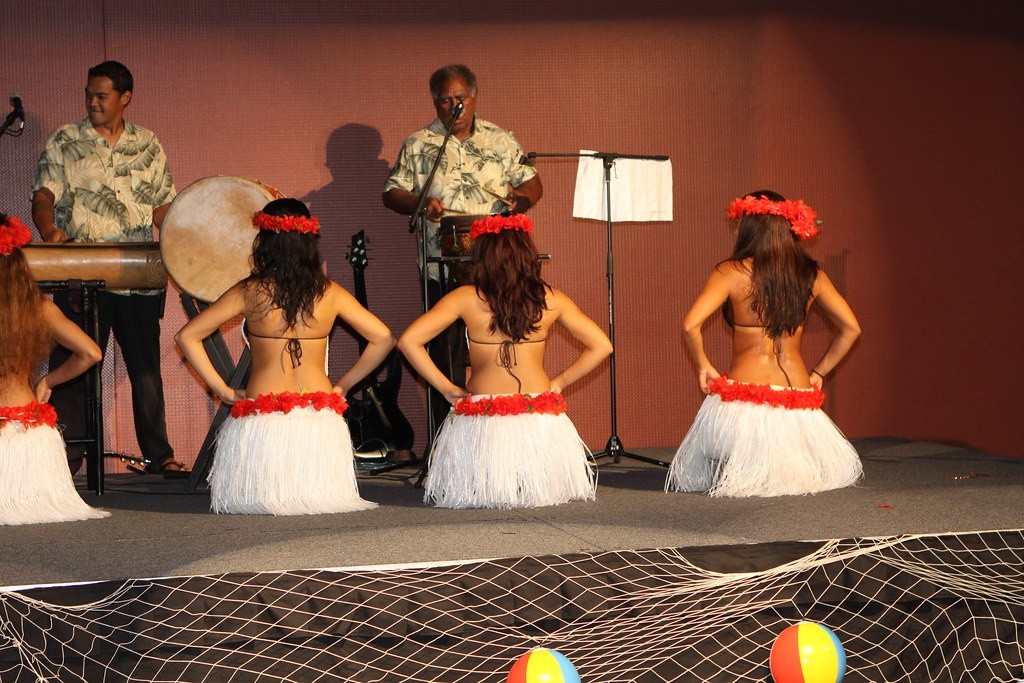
[812,370,825,378]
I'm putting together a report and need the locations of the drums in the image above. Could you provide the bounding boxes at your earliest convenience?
[159,174,287,305]
[441,215,494,257]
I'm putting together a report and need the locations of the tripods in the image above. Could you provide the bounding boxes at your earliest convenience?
[573,159,673,468]
[369,206,435,487]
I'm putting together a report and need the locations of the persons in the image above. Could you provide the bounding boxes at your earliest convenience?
[381,64,543,436]
[0,211,112,526]
[397,211,613,510]
[30,60,191,473]
[173,197,397,516]
[664,190,863,499]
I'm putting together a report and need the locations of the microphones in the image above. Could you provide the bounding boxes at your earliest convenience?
[9,91,25,122]
[451,102,466,117]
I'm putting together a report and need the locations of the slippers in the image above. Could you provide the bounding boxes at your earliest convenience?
[145,462,189,471]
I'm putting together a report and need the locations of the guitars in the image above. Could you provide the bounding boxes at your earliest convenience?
[342,229,415,464]
